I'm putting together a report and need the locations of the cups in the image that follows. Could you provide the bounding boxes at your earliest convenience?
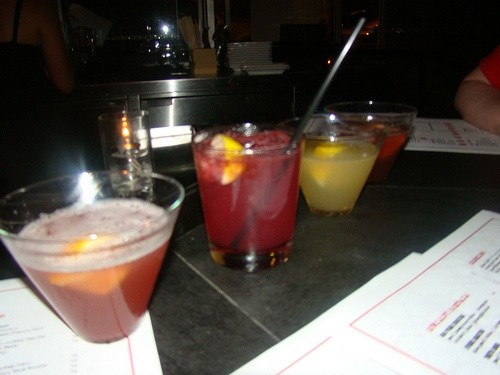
[191,125,301,272]
[279,122,386,219]
[324,101,416,183]
[97,110,155,203]
[0,170,186,343]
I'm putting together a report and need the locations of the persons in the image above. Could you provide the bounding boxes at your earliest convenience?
[455,47,500,138]
[1,0,75,197]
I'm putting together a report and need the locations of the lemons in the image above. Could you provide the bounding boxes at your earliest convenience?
[310,144,347,185]
[212,134,246,185]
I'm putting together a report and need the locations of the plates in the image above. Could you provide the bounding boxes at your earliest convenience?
[227,42,290,75]
